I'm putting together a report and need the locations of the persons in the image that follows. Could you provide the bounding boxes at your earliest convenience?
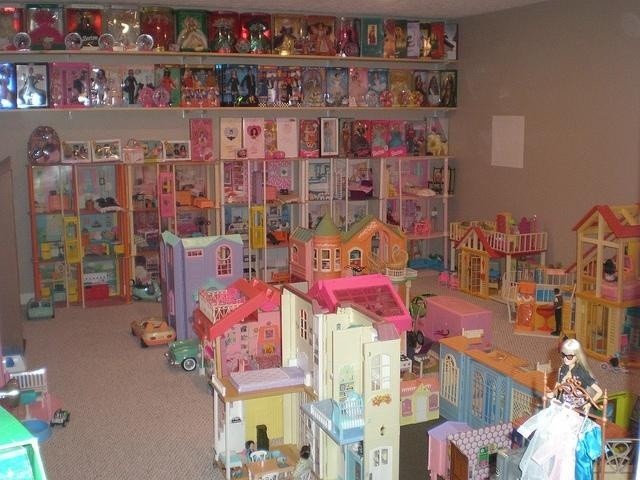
[0,1,456,263]
[604,260,617,282]
[246,441,310,480]
[549,288,563,336]
[513,336,609,480]
